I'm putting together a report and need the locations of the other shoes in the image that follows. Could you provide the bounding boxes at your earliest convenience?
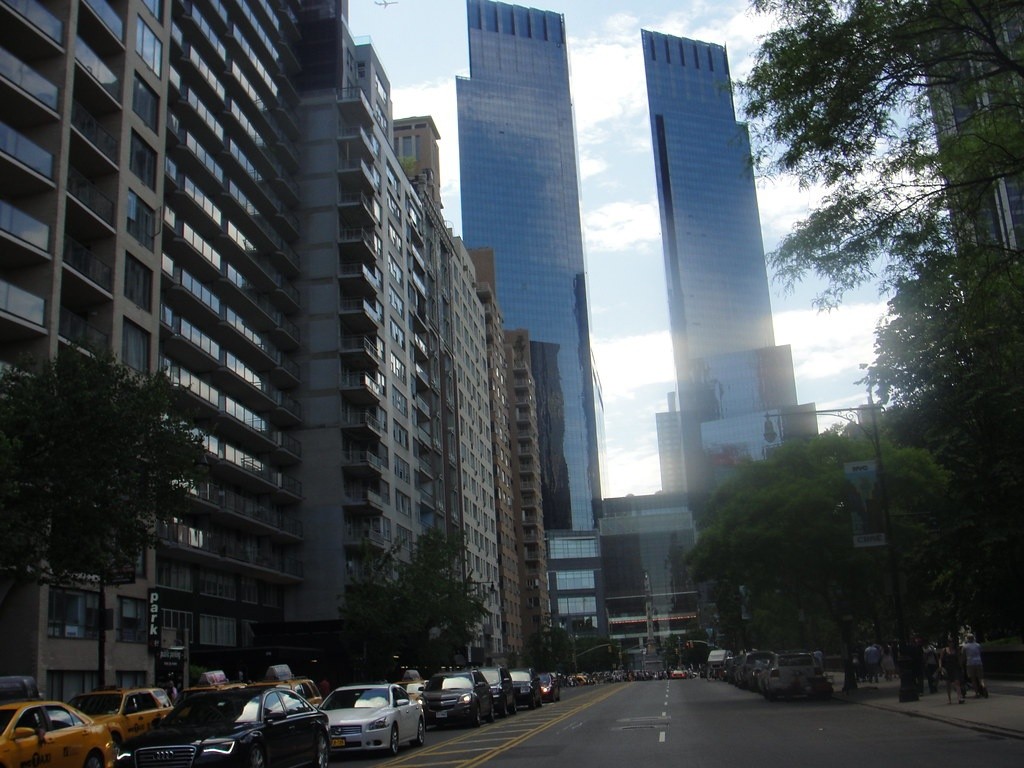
[959,700,965,704]
[984,690,988,698]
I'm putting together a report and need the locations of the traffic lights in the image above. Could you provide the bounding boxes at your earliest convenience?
[686,641,691,648]
[674,648,679,654]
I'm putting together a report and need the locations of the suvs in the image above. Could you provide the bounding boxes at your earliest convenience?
[418,668,495,728]
[469,665,517,719]
[506,667,542,710]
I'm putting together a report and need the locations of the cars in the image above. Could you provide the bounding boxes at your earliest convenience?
[537,671,560,703]
[700,646,823,703]
[113,686,332,768]
[170,669,248,723]
[244,664,326,710]
[671,670,684,679]
[313,684,426,757]
[0,701,117,768]
[391,670,429,705]
[68,685,174,757]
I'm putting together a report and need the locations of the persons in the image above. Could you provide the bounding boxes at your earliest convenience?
[910,625,988,705]
[167,680,177,699]
[853,640,900,683]
[561,663,707,688]
[317,676,330,697]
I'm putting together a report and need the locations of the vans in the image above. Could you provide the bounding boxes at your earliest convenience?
[0,676,44,708]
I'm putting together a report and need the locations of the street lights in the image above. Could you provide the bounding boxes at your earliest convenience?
[763,396,920,703]
